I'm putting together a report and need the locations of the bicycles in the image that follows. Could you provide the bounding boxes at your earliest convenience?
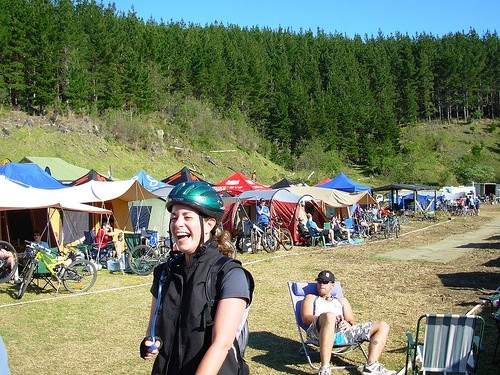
[383,211,401,239]
[0,239,19,284]
[261,215,294,253]
[224,240,237,260]
[249,219,278,254]
[127,227,179,276]
[16,239,98,300]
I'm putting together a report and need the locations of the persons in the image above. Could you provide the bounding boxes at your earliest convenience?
[0,181,500,375]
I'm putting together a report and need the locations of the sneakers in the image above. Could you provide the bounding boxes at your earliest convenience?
[361,361,397,375]
[318,366,331,375]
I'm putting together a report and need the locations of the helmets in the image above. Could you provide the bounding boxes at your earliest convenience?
[165,180,225,225]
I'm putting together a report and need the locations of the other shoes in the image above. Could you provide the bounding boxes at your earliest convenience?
[332,242,341,247]
[347,241,354,245]
[14,278,23,284]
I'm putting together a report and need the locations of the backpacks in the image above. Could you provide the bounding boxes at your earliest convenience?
[205,256,250,365]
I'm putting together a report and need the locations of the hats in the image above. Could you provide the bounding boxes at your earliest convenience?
[315,270,335,283]
[260,198,266,202]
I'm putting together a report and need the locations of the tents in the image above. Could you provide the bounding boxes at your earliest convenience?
[0,155,440,267]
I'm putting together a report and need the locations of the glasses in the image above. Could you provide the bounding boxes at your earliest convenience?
[316,279,331,284]
[33,234,40,237]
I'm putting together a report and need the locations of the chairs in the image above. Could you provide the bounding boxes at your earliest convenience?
[125,233,158,272]
[344,218,364,236]
[84,230,114,262]
[33,247,60,295]
[324,222,343,237]
[241,221,260,254]
[296,229,310,247]
[287,280,368,371]
[308,227,326,247]
[404,313,485,375]
